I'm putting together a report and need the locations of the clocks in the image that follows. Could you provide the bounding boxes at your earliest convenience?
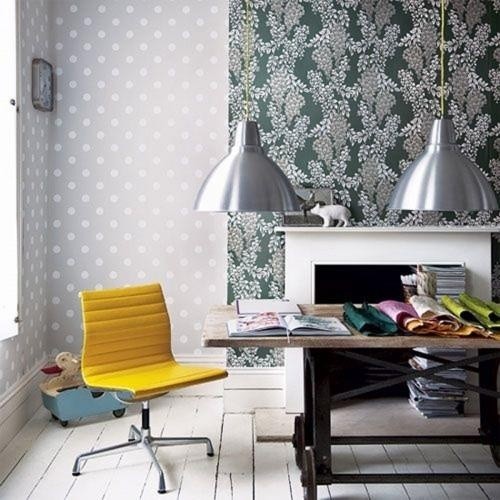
[33,59,53,112]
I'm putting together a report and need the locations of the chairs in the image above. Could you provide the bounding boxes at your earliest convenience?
[71,283,227,493]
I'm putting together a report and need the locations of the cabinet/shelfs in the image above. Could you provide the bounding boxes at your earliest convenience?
[274,226,498,414]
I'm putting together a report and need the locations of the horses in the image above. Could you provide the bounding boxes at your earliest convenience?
[297,195,326,225]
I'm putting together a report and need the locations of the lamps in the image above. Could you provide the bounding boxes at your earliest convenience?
[388,1,497,211]
[193,0,297,212]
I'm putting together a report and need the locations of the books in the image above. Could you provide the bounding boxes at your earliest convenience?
[225,314,353,337]
[236,299,302,318]
[408,264,470,420]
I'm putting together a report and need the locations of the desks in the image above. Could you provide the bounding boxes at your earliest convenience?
[203,304,499,499]
